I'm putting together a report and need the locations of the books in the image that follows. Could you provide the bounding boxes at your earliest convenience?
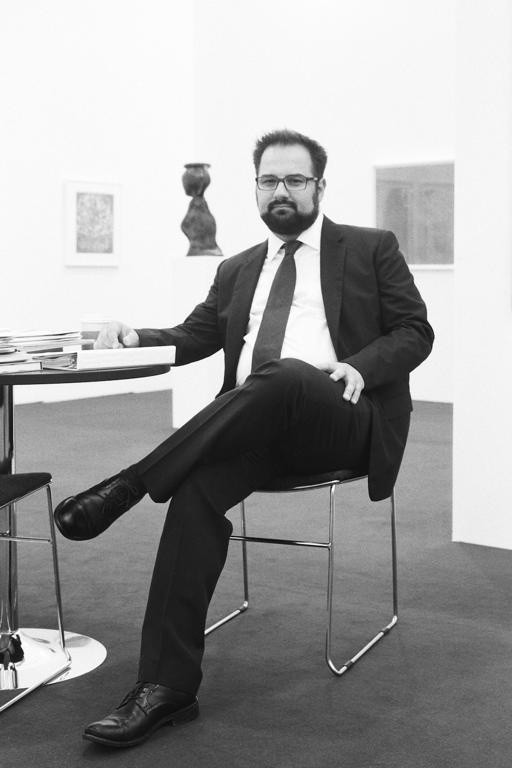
[0,328,178,374]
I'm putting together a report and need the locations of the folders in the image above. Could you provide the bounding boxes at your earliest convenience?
[34,345,176,371]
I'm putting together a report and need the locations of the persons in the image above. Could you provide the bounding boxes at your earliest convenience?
[52,129,435,749]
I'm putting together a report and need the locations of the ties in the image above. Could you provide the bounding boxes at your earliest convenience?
[252,240,302,373]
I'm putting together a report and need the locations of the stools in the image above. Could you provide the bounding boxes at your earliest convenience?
[201,470,402,675]
[1,469,77,714]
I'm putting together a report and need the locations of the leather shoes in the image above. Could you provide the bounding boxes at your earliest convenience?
[53,471,145,541]
[82,685,199,748]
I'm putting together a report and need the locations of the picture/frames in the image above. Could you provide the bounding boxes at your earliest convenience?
[374,160,456,273]
[63,180,127,269]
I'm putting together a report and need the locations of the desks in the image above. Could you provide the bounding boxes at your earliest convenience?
[0,331,175,689]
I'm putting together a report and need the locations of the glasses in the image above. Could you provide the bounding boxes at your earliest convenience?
[255,174,318,191]
